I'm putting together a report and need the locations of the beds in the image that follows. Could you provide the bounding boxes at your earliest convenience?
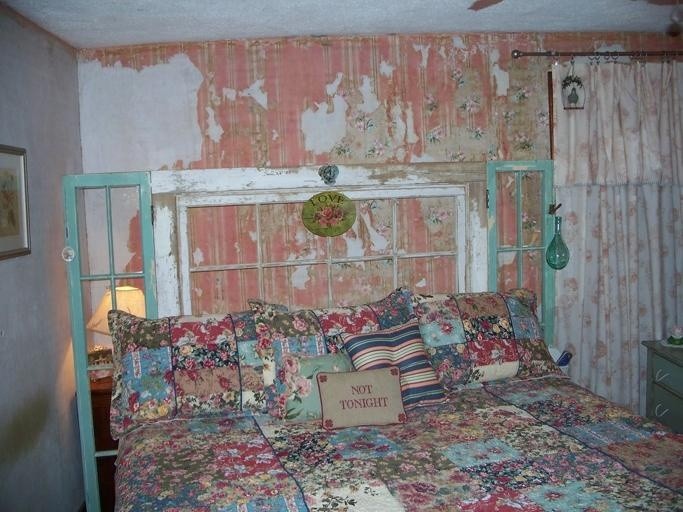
[92,285,682,512]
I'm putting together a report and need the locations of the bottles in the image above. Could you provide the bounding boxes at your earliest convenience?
[545,215,569,270]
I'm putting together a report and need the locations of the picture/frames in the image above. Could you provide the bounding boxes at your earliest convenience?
[0,145,32,261]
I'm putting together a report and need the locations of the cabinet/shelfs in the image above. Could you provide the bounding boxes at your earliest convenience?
[636,334,682,434]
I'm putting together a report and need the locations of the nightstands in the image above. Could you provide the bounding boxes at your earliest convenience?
[82,369,121,511]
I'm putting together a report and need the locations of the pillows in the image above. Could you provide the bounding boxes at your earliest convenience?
[340,314,452,416]
[241,287,420,418]
[412,288,571,394]
[313,365,407,433]
[104,305,269,443]
[277,347,352,425]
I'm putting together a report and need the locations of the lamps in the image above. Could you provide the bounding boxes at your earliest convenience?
[77,284,149,340]
[545,202,568,269]
